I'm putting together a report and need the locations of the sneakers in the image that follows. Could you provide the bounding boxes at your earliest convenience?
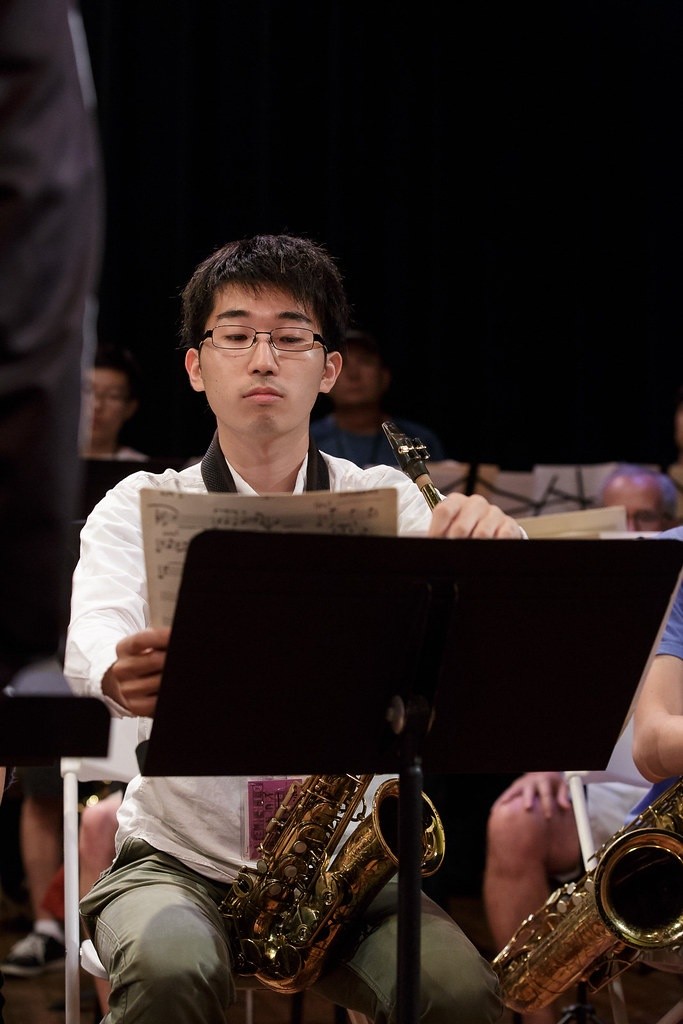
[0,932,65,976]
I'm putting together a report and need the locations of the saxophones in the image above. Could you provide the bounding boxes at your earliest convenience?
[486,781,683,1015]
[214,422,448,997]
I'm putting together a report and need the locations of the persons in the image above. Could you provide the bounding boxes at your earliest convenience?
[0,233,683,1024]
[0,0,95,676]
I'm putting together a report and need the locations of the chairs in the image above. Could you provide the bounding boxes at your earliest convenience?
[59,757,260,1024]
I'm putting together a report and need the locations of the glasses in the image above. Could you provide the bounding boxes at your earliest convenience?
[198,325,331,352]
[628,511,673,524]
